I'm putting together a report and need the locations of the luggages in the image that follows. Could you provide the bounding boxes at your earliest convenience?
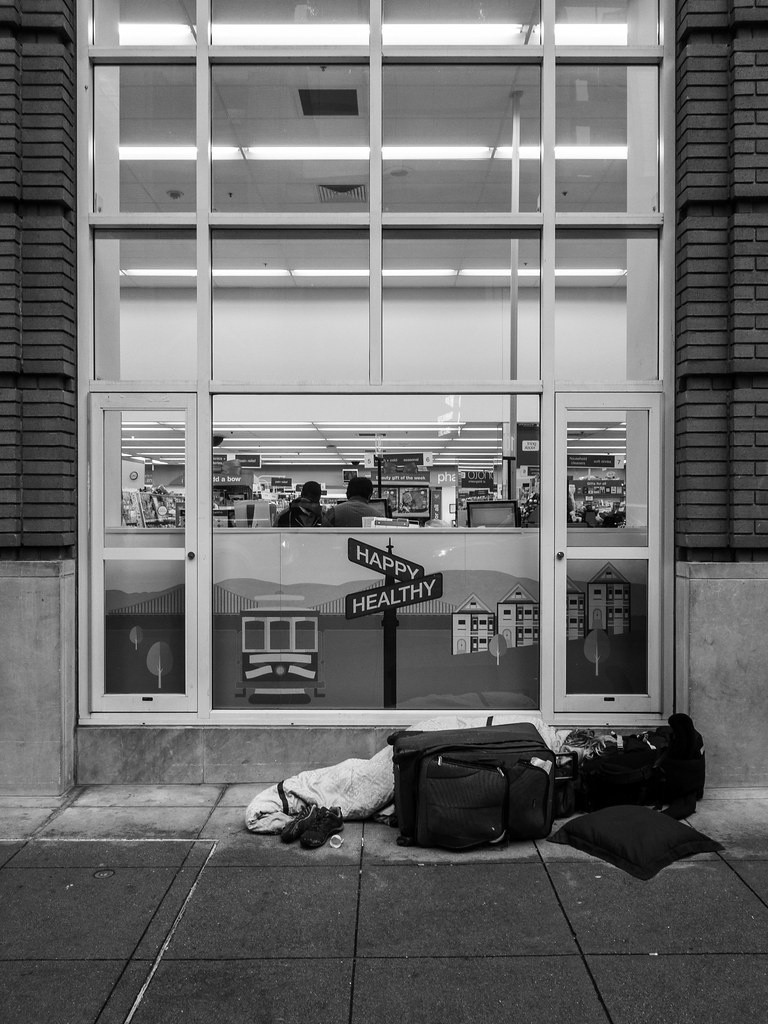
[386,722,579,850]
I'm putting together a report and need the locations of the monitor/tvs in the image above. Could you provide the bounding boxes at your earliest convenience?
[342,469,358,482]
[467,500,521,527]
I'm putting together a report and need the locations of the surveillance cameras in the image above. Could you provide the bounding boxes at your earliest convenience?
[213,437,225,446]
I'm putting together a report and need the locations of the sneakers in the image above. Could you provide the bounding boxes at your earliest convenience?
[280,802,319,842]
[299,805,344,847]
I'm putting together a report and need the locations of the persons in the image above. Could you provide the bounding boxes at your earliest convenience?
[272,481,334,528]
[245,711,601,835]
[321,475,387,528]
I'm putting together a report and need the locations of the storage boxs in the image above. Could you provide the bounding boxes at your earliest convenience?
[385,722,557,848]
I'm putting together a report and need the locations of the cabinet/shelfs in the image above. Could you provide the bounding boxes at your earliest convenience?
[121,477,626,528]
